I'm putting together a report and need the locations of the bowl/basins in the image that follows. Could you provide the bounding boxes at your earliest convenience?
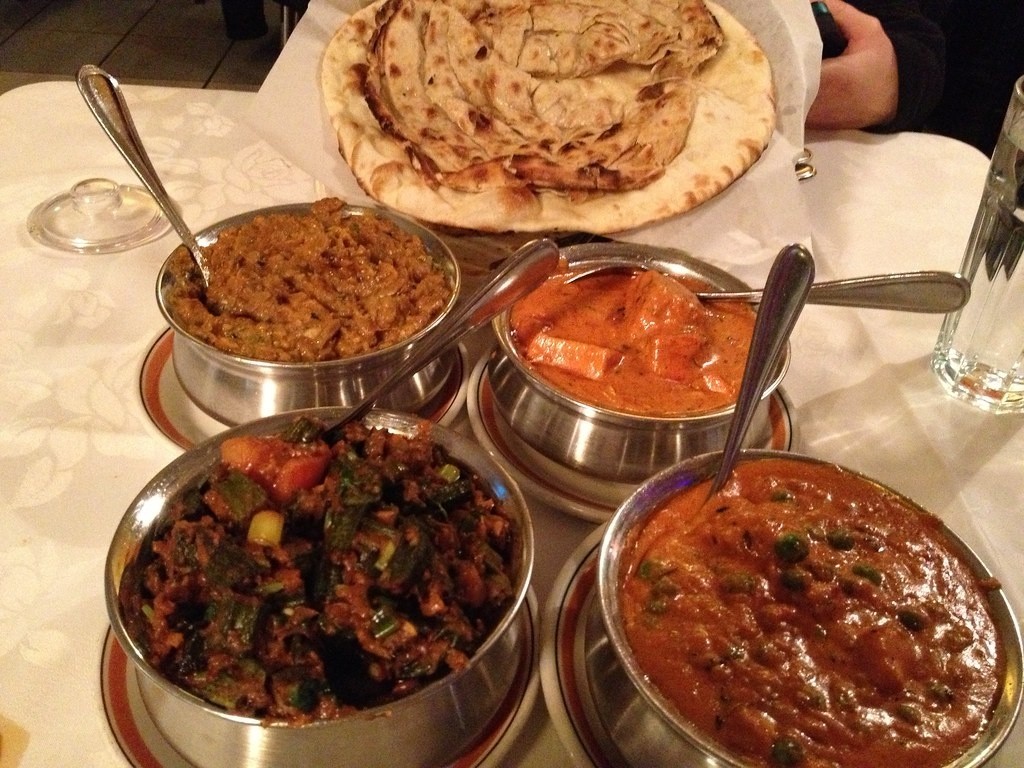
[491,243,790,503]
[157,204,460,429]
[104,409,534,768]
[598,451,1023,768]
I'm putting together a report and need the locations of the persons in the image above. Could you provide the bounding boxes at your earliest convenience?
[804,1,945,133]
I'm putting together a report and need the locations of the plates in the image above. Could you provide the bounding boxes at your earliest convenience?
[99,588,539,768]
[138,323,468,451]
[467,347,798,522]
[542,519,605,768]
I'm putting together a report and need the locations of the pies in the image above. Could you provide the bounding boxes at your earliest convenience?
[321,1,779,229]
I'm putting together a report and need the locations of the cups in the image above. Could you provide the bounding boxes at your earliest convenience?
[931,77,1024,417]
